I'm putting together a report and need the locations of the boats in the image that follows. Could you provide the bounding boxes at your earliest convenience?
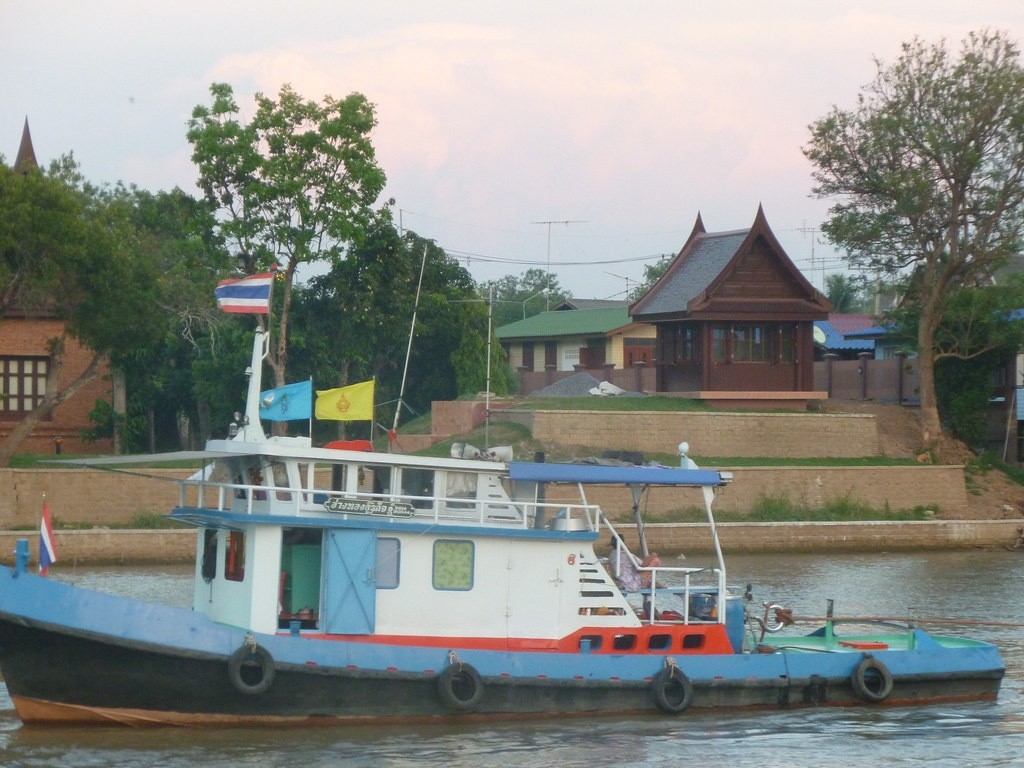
[0,236,1008,733]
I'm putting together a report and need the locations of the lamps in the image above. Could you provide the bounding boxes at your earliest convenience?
[857,366,861,373]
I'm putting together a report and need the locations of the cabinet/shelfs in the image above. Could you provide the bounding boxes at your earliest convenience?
[279,544,321,613]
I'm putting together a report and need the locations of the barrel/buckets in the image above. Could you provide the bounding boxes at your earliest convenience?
[713,594,745,654]
[687,592,712,622]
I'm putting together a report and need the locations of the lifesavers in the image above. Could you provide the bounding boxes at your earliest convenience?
[228,644,275,695]
[438,661,486,712]
[852,660,895,704]
[650,666,692,715]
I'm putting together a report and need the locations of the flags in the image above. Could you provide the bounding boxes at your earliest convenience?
[215,272,273,313]
[315,380,374,420]
[39,505,60,576]
[260,380,311,421]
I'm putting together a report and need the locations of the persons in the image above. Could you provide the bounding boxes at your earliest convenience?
[606,534,667,592]
